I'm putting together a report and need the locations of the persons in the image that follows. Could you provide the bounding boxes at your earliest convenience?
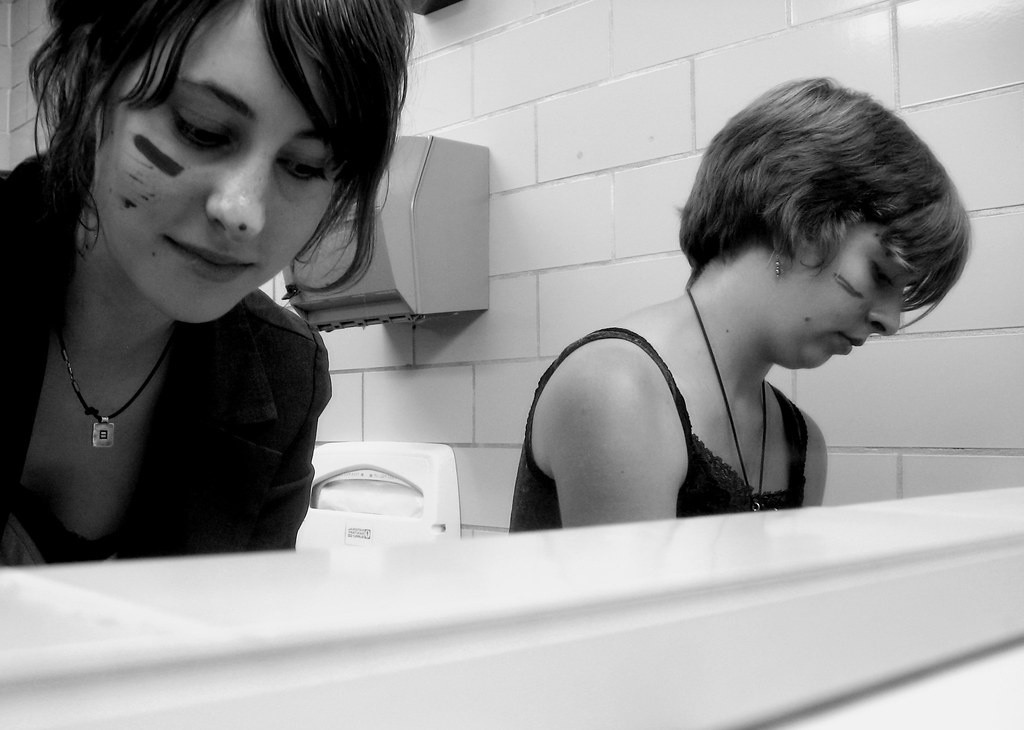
[0,0,412,573]
[507,80,973,534]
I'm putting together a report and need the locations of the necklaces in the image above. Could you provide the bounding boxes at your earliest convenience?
[686,286,767,512]
[60,330,176,449]
